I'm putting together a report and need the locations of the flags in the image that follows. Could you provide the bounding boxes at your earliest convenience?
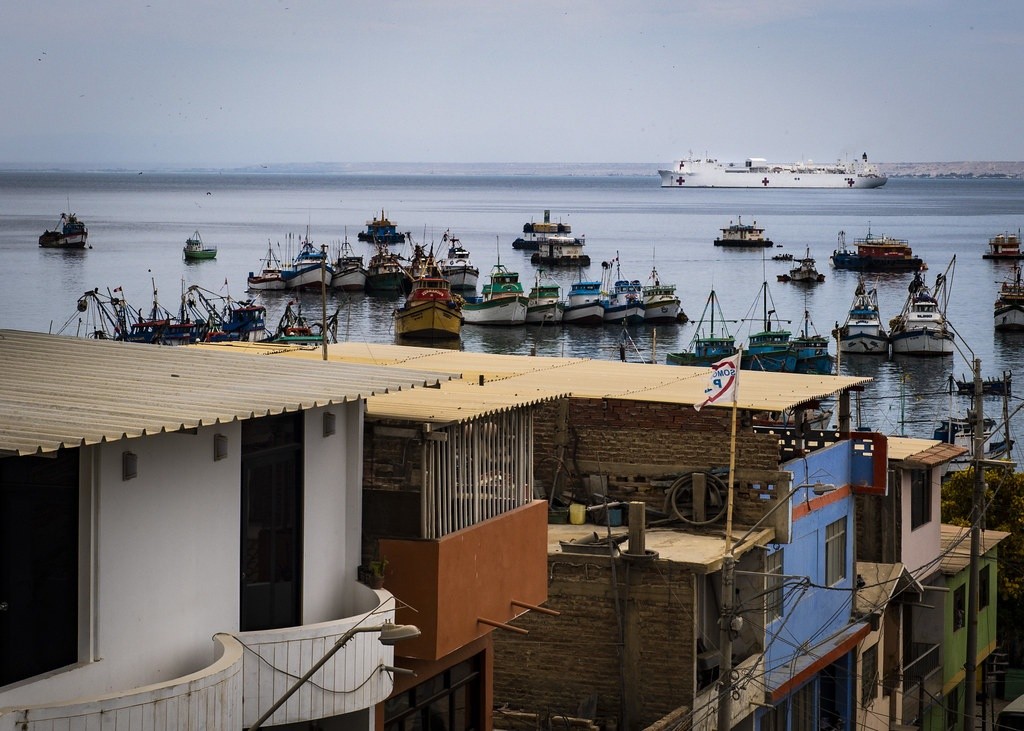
[694,352,739,412]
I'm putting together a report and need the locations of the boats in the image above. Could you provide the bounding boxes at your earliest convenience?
[526,253,565,326]
[713,215,774,248]
[460,234,530,326]
[657,150,888,190]
[390,226,465,340]
[641,243,681,325]
[888,252,957,357]
[599,250,646,326]
[182,229,218,260]
[48,208,480,350]
[829,220,929,275]
[733,246,836,375]
[357,208,406,245]
[993,261,1024,333]
[666,263,738,367]
[511,209,592,269]
[38,212,89,250]
[982,227,1024,260]
[831,273,888,355]
[933,408,1016,473]
[789,243,819,282]
[955,376,1012,397]
[562,263,606,325]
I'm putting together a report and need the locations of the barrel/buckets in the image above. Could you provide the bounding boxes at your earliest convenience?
[602,508,623,526]
[569,503,587,525]
